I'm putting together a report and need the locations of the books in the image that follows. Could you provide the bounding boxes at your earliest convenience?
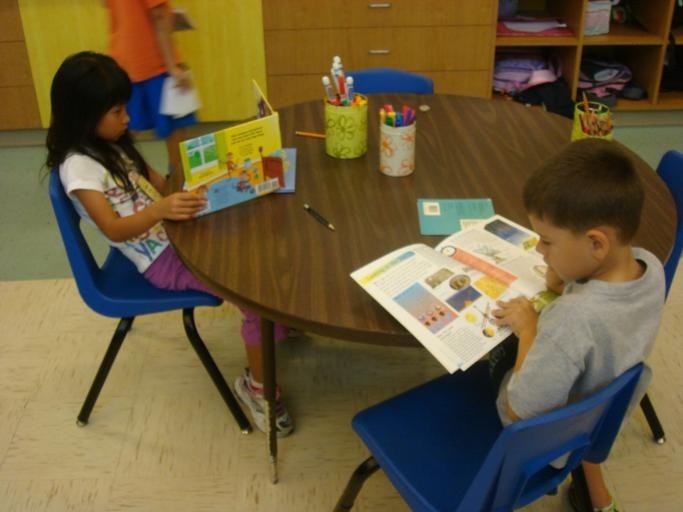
[272,148,297,194]
[417,198,496,236]
[350,213,549,377]
[179,112,284,219]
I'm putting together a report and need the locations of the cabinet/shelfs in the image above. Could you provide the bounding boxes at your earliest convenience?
[491,3,680,113]
[0,1,266,129]
[260,2,493,107]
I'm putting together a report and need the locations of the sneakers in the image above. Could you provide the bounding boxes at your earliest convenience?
[234,365,295,438]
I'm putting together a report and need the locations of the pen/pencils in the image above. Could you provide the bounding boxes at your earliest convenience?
[380,105,417,125]
[320,56,367,104]
[304,204,336,231]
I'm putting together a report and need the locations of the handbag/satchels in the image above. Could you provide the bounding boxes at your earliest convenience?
[493,53,574,120]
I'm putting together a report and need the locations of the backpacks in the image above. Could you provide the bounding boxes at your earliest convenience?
[579,58,645,107]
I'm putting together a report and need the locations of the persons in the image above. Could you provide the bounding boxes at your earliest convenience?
[474,137,666,512]
[106,0,198,180]
[46,51,304,439]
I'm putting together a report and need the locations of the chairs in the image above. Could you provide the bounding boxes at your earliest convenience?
[328,358,645,512]
[45,166,256,437]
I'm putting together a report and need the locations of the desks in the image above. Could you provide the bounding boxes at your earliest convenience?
[169,93,682,483]
